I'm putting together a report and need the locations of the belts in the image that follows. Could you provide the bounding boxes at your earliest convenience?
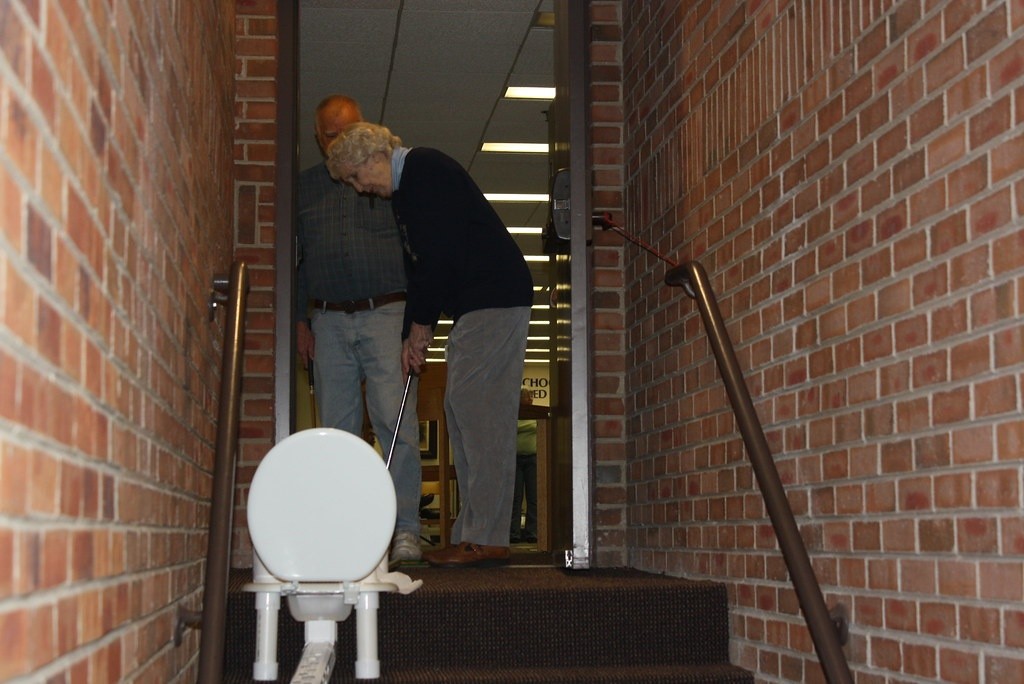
[310,292,408,313]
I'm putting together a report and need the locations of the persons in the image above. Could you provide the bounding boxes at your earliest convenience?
[325,122,534,564]
[295,95,423,561]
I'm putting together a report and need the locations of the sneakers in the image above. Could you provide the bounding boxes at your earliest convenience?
[392,532,422,560]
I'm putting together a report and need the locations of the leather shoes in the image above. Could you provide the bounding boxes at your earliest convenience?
[422,542,510,567]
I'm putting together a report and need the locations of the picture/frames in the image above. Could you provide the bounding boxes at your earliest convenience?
[419,421,438,458]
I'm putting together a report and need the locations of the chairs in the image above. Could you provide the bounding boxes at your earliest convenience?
[417,362,457,551]
